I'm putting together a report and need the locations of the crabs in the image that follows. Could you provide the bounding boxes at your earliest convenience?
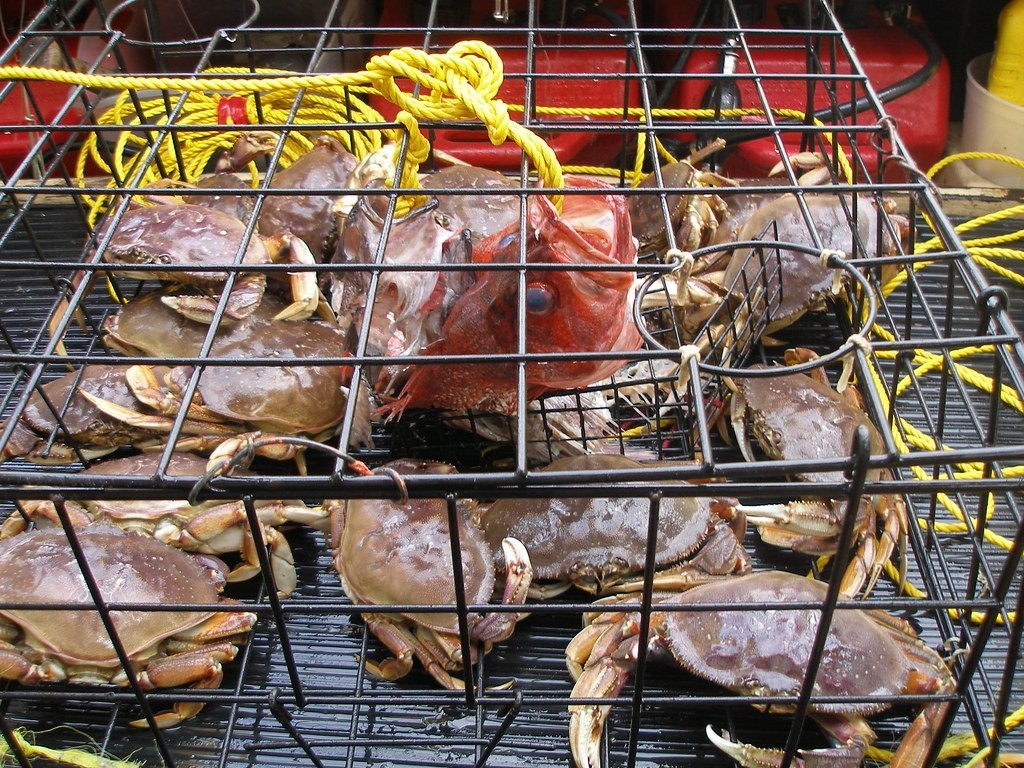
[4,133,531,768]
[478,136,955,768]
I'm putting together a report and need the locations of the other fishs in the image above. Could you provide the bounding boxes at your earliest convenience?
[330,176,645,424]
